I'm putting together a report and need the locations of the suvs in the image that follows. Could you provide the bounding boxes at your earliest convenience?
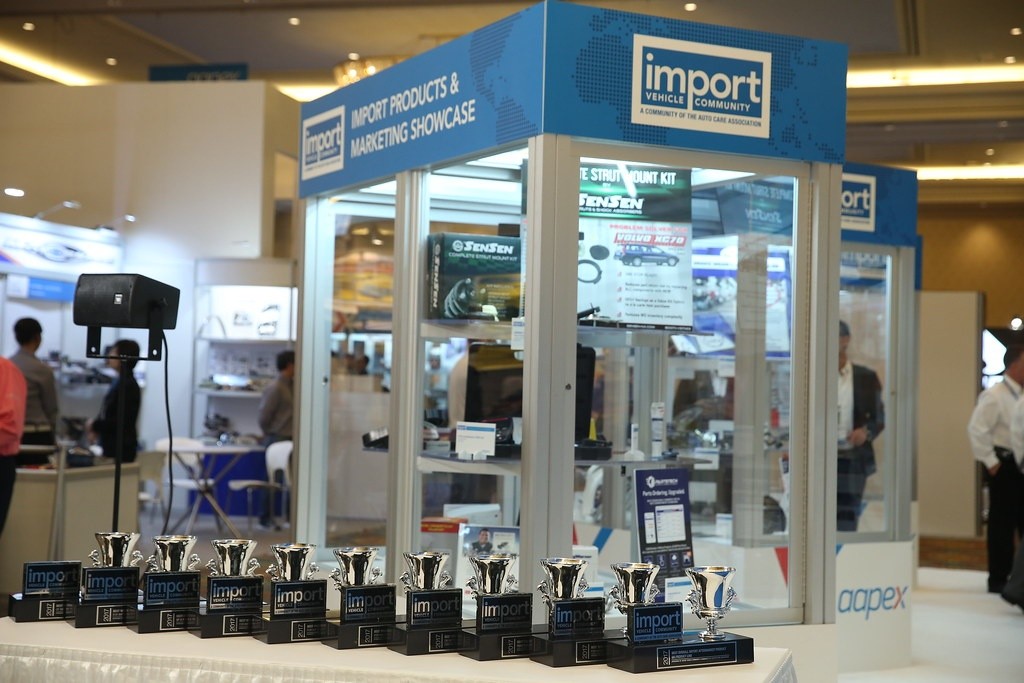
[613,244,679,266]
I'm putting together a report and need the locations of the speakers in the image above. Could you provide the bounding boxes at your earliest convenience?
[73,273,181,328]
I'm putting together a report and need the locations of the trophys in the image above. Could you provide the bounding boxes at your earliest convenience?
[329,547,384,592]
[536,557,590,610]
[684,566,737,639]
[399,551,452,594]
[88,532,144,567]
[146,534,200,572]
[608,562,660,634]
[466,553,518,600]
[205,539,261,577]
[266,542,319,590]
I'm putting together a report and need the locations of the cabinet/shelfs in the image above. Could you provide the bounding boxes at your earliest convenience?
[190,338,300,523]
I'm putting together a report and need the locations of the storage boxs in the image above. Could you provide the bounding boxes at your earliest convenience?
[5,559,755,674]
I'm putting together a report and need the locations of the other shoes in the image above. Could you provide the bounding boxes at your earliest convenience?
[258,522,274,532]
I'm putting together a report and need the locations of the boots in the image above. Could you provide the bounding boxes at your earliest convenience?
[1001,539,1024,611]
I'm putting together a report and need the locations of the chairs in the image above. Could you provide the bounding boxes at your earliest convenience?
[157,439,220,537]
[225,439,298,537]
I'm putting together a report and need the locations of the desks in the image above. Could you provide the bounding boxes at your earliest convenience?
[158,445,265,550]
[365,445,665,554]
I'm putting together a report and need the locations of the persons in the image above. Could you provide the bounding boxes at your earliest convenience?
[256,350,295,531]
[0,355,26,535]
[9,317,59,464]
[85,339,142,463]
[426,354,450,429]
[471,528,492,552]
[330,350,368,375]
[837,320,886,532]
[968,344,1024,610]
